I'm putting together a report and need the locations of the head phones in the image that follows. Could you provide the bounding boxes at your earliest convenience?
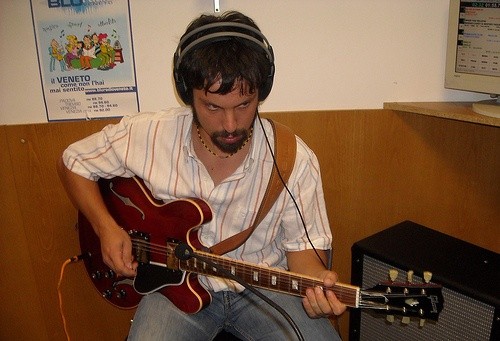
[172,21,275,105]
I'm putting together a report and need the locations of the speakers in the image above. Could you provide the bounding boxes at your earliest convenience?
[347,220,500,341]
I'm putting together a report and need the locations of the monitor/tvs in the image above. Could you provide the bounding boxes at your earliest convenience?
[444,0,500,119]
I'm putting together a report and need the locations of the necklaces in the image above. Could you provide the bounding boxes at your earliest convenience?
[196,120,255,159]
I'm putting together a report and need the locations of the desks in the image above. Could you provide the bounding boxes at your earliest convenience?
[384,102,500,127]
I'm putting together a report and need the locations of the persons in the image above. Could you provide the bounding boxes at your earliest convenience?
[61,10,346,341]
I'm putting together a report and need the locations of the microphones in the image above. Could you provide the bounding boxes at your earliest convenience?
[175,243,304,341]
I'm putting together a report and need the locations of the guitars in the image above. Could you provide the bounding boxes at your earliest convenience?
[77,174,445,328]
